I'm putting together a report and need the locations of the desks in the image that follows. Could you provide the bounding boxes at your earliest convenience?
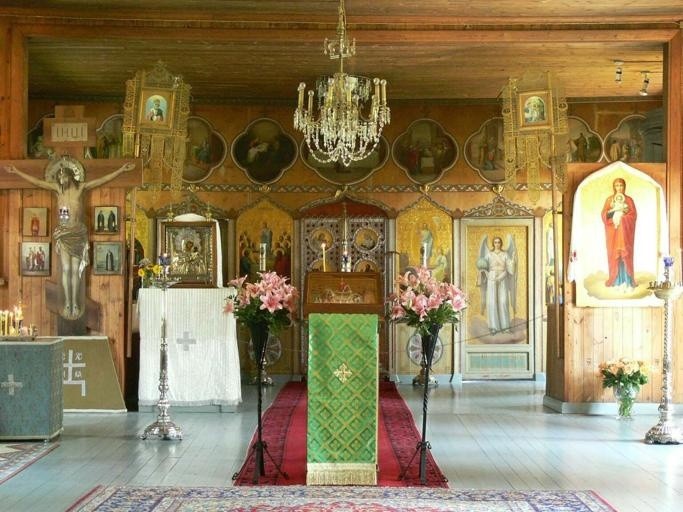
[2,336,65,447]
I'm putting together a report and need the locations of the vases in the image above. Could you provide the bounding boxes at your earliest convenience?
[614,383,637,417]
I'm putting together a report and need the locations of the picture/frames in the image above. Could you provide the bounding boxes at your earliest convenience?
[158,220,216,290]
[91,201,120,235]
[137,86,176,132]
[517,90,553,132]
[21,207,50,238]
[90,239,123,278]
[17,241,51,279]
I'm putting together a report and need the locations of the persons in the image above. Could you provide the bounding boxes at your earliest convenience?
[475,236,518,335]
[24,246,35,271]
[2,160,138,322]
[566,128,644,163]
[104,248,113,272]
[193,135,210,166]
[401,139,452,176]
[419,221,435,269]
[609,192,628,230]
[435,242,451,273]
[170,238,205,275]
[29,213,38,236]
[94,209,104,232]
[314,232,329,250]
[84,130,124,159]
[35,246,44,271]
[238,220,292,285]
[144,98,164,121]
[107,209,116,232]
[360,232,375,250]
[477,140,501,170]
[244,134,272,165]
[600,177,639,289]
[358,263,374,272]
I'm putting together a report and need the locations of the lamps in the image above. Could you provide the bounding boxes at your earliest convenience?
[613,58,627,85]
[293,3,391,167]
[635,68,652,97]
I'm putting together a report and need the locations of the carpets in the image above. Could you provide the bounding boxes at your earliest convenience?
[0,438,54,484]
[61,482,612,512]
[232,378,448,483]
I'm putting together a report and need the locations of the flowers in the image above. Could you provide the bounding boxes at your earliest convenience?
[382,266,464,336]
[131,255,174,282]
[221,265,297,331]
[600,358,647,385]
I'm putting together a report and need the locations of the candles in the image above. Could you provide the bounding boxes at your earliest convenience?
[320,242,325,271]
